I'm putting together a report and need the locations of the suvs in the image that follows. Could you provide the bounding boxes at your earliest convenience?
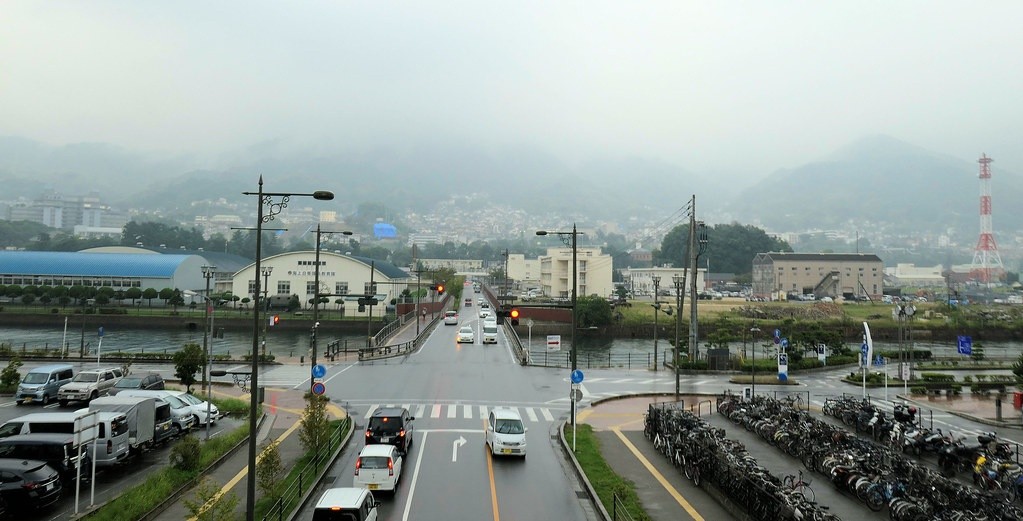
[103,372,167,398]
[0,457,65,518]
[57,367,123,407]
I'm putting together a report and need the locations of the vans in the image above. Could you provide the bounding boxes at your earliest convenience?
[312,487,382,521]
[0,412,134,464]
[17,364,75,406]
[445,311,459,325]
[120,389,195,430]
[485,406,529,458]
[352,444,403,496]
[156,400,175,445]
[0,436,90,486]
[364,407,415,458]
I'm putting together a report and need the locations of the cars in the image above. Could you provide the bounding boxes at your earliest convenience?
[786,289,928,305]
[473,282,485,294]
[465,297,473,306]
[477,297,491,317]
[456,327,477,343]
[612,288,766,303]
[169,391,220,428]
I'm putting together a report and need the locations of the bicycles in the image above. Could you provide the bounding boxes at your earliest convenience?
[643,391,1023,521]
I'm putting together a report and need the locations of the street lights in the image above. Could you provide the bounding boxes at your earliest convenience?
[650,274,662,371]
[209,172,337,521]
[750,319,762,401]
[536,223,599,426]
[199,264,216,391]
[261,265,278,358]
[307,224,354,418]
[670,272,685,401]
[183,289,213,442]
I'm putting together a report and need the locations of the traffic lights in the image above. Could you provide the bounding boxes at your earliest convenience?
[662,307,673,316]
[438,286,444,296]
[568,349,572,362]
[651,303,661,310]
[496,310,511,317]
[258,386,265,402]
[430,284,439,290]
[511,309,520,327]
[270,314,280,327]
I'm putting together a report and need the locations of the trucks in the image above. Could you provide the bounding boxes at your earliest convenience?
[75,396,162,455]
[483,317,499,344]
